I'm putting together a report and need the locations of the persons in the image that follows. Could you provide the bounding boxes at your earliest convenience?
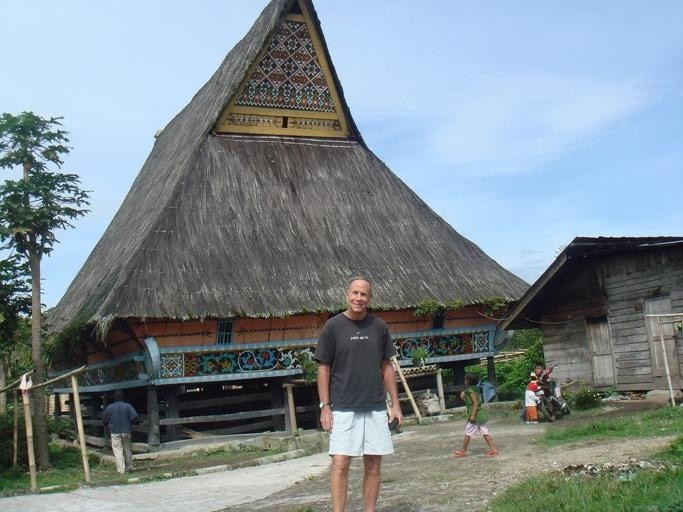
[311,275,404,512]
[102,389,141,474]
[524,382,542,424]
[521,363,550,421]
[454,371,499,458]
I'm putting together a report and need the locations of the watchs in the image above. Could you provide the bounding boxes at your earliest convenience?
[318,402,330,408]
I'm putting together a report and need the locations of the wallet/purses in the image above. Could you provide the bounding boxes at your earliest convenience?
[389,417,400,430]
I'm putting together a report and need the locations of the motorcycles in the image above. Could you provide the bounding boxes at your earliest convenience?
[535,362,571,422]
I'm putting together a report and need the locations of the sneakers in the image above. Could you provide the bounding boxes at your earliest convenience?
[454,451,465,456]
[487,449,499,456]
